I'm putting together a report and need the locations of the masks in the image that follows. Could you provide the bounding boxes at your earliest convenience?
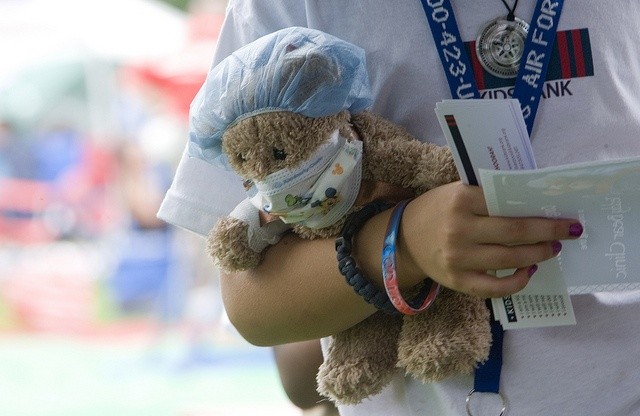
[241,126,364,229]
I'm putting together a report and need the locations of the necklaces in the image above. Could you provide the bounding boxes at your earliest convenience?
[478,0,533,80]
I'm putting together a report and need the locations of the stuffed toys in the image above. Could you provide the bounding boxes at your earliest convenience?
[189,23,494,407]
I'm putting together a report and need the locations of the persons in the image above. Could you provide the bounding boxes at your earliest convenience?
[154,2,637,412]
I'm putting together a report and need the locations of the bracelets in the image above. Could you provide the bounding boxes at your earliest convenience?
[381,196,440,316]
[334,200,432,313]
